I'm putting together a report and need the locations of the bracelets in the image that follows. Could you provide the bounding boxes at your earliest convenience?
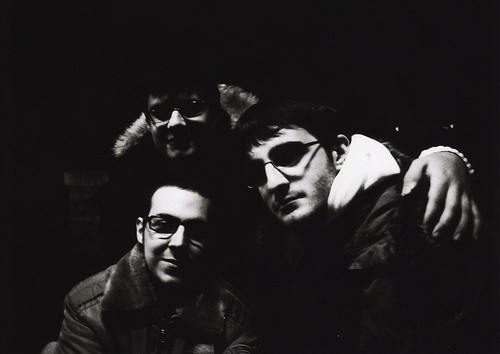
[420,140,478,180]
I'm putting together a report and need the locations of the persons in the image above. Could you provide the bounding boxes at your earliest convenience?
[55,56,482,274]
[39,181,270,353]
[223,93,499,354]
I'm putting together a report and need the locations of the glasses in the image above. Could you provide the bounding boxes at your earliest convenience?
[145,97,211,122]
[142,214,212,240]
[238,140,322,190]
